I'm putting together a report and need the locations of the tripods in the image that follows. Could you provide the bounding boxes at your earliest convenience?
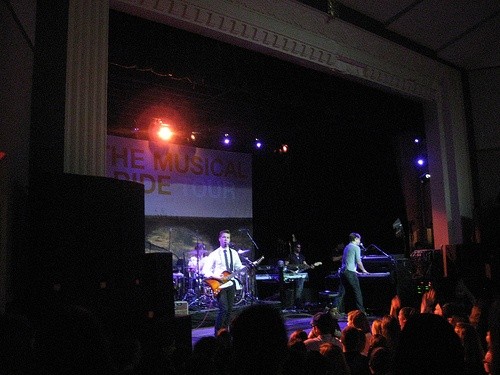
[173,238,217,312]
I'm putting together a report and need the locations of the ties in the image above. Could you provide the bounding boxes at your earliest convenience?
[224,250,230,272]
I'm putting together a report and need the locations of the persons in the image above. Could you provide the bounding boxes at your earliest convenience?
[188,243,206,267]
[0,289,500,375]
[288,243,315,307]
[334,233,369,316]
[202,230,245,336]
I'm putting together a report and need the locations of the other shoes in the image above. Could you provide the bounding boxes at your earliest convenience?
[362,312,369,316]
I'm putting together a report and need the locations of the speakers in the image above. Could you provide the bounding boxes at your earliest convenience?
[411,242,496,319]
[17,166,193,363]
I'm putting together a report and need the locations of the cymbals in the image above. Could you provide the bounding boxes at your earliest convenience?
[186,250,208,254]
[236,249,252,254]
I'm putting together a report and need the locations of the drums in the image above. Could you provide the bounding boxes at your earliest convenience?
[232,289,245,305]
[185,267,197,290]
[199,282,213,296]
[173,273,185,289]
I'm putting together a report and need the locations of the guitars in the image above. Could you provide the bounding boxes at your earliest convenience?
[282,262,322,283]
[202,256,264,299]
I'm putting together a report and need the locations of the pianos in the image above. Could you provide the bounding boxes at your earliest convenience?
[327,255,405,315]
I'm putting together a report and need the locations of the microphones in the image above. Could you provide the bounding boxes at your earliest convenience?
[360,243,367,251]
[238,229,249,231]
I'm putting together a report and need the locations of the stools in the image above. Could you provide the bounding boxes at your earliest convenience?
[319,290,341,322]
